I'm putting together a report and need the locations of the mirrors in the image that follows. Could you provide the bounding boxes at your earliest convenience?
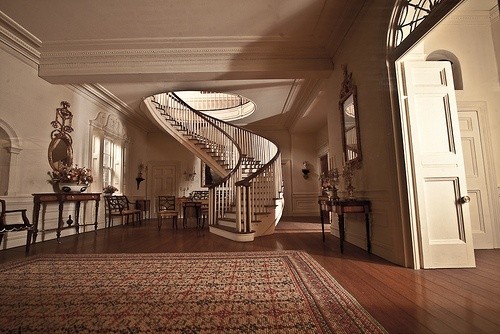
[338,63,362,170]
[48,101,74,172]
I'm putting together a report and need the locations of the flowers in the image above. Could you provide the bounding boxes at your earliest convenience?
[46,161,93,185]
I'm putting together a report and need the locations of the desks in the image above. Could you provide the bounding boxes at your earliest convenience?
[193,199,230,229]
[32,193,102,245]
[318,196,371,254]
[136,200,150,222]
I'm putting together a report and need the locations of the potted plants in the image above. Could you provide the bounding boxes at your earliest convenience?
[103,185,118,195]
[320,167,356,199]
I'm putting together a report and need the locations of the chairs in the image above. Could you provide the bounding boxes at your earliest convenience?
[182,201,202,229]
[0,199,34,256]
[116,195,141,226]
[104,196,133,231]
[158,195,178,230]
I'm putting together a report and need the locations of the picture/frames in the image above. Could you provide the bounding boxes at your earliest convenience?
[201,159,230,187]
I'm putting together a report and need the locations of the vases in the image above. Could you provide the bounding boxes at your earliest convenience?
[59,183,88,193]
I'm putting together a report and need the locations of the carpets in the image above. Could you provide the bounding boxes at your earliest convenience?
[0,250,374,334]
